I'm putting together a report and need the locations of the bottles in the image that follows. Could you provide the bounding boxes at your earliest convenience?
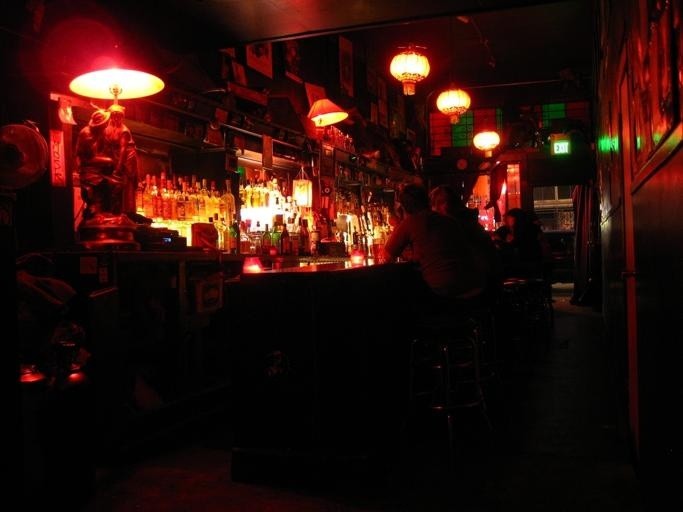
[132,167,402,267]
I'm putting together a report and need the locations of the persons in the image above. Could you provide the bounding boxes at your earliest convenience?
[105,104,138,213]
[71,108,112,214]
[499,208,553,280]
[430,184,498,270]
[374,185,481,300]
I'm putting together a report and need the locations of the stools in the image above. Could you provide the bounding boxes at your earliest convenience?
[405,276,562,453]
